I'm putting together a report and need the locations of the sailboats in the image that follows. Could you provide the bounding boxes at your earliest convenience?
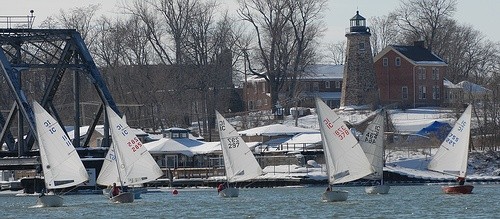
[95,113,142,200]
[128,184,148,196]
[214,108,265,199]
[313,92,377,203]
[361,107,390,195]
[29,90,91,207]
[106,104,165,203]
[423,103,475,195]
